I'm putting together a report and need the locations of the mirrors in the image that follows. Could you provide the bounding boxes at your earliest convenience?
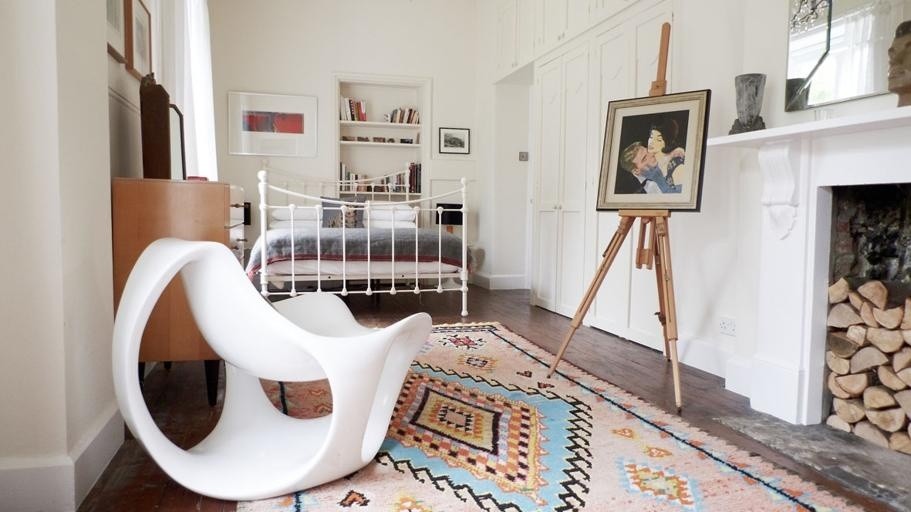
[785,0,911,112]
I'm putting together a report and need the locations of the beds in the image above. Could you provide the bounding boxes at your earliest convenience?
[255,159,469,317]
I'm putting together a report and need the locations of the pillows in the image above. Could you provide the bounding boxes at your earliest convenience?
[271,195,416,229]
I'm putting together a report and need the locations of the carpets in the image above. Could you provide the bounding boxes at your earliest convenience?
[235,320,866,511]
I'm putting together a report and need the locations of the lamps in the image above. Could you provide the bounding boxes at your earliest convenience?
[434,203,463,235]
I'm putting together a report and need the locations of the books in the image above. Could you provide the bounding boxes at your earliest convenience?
[340,135,369,142]
[340,160,420,193]
[340,95,367,122]
[383,106,419,125]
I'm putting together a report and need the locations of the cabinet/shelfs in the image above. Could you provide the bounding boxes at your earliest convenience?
[333,70,430,229]
[111,177,248,406]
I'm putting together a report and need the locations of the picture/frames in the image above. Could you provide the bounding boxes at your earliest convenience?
[225,90,318,158]
[438,126,471,154]
[106,0,152,80]
[596,89,710,213]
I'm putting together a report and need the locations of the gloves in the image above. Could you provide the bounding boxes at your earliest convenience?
[638,163,682,193]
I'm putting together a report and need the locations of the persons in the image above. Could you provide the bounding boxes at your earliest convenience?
[621,116,685,193]
[888,20,911,107]
